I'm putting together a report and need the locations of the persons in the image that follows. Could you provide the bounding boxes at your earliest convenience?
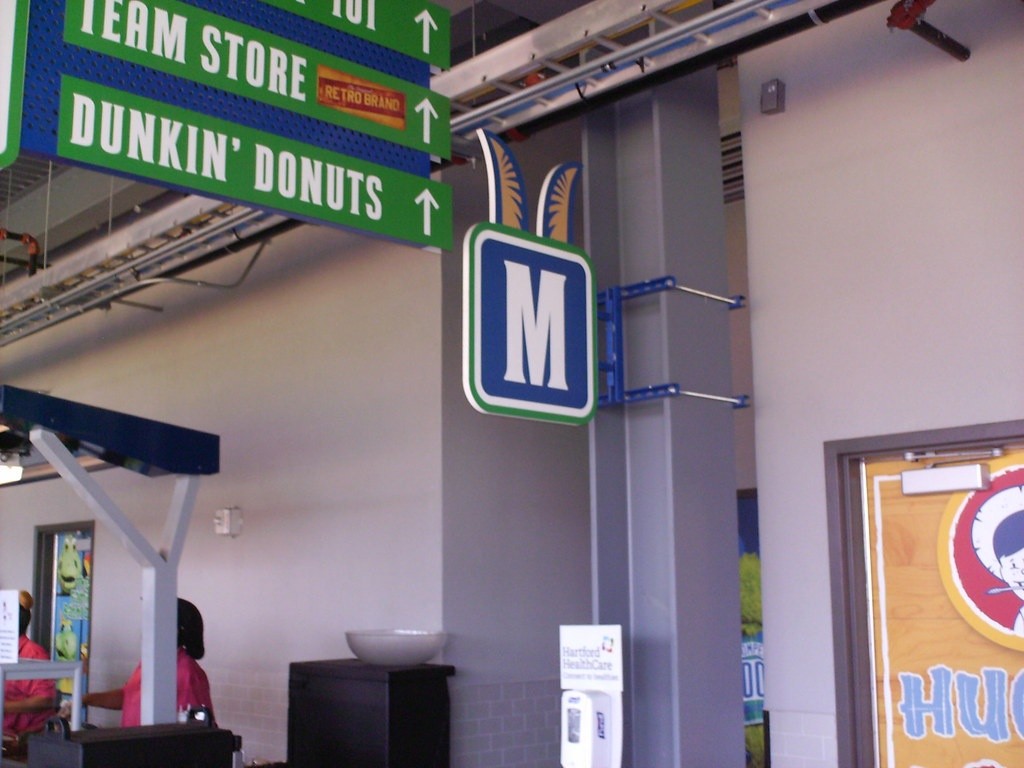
[2,602,59,763]
[54,598,218,732]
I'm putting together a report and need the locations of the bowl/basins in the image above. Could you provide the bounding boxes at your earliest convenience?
[344,629,447,665]
